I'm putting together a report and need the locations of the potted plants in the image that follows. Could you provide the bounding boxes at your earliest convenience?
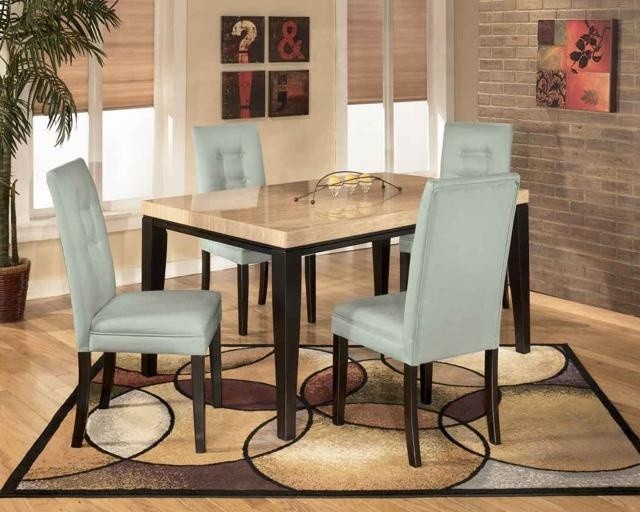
[0,1,127,324]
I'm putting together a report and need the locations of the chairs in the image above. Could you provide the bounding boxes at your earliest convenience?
[192,123,316,337]
[332,172,520,472]
[45,157,222,455]
[398,119,514,310]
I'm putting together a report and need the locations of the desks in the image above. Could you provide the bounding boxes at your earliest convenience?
[140,170,530,440]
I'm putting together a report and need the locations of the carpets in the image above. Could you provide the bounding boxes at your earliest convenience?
[0,343,640,498]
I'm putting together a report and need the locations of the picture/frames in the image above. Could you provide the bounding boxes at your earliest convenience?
[268,15,311,63]
[220,14,265,63]
[269,70,309,116]
[537,19,613,113]
[223,70,266,119]
